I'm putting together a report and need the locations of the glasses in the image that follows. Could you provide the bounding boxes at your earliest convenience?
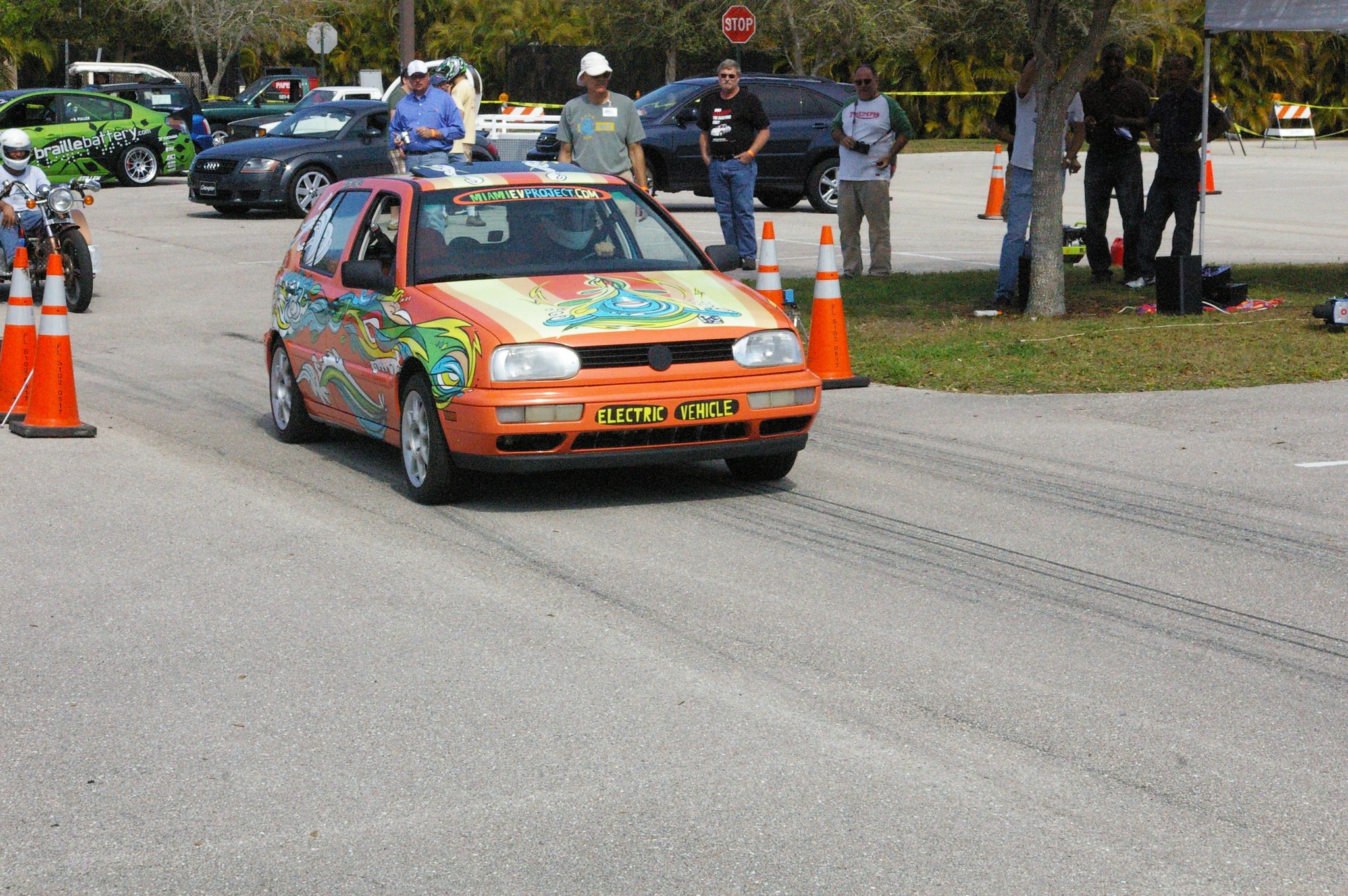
[718,73,738,78]
[588,72,609,79]
[854,78,875,85]
[410,72,427,80]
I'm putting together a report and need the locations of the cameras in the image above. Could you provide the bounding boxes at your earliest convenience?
[850,141,869,154]
[396,131,410,144]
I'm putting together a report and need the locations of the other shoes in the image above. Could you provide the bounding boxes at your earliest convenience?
[738,256,756,271]
[1091,269,1113,282]
[840,273,852,279]
[387,218,399,230]
[1121,274,1136,283]
[8,262,14,273]
[987,295,1012,312]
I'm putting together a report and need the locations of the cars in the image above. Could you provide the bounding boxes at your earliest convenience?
[0,61,214,189]
[188,99,502,218]
[263,159,821,507]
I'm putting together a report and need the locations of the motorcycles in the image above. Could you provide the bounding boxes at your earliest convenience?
[0,179,102,313]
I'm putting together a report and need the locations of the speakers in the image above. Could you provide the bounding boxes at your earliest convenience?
[1154,255,1202,316]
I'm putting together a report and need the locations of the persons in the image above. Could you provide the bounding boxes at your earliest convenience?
[987,48,1085,306]
[1124,55,1232,290]
[1070,43,1154,286]
[828,63,913,279]
[385,55,487,238]
[556,51,651,247]
[697,58,771,270]
[0,127,65,275]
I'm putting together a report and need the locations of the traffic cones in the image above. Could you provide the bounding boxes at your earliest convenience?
[1,246,98,439]
[803,225,870,390]
[1197,147,1223,196]
[977,142,1006,221]
[755,221,783,311]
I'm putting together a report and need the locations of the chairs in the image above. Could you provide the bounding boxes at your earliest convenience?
[384,227,462,279]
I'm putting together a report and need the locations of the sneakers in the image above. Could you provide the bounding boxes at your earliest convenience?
[466,212,486,226]
[1125,276,1156,288]
[443,216,448,227]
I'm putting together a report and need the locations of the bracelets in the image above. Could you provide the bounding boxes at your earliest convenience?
[639,186,649,191]
[1074,154,1078,158]
[747,149,754,157]
[431,129,435,137]
[1065,157,1071,164]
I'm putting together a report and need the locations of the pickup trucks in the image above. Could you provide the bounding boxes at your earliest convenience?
[198,74,382,140]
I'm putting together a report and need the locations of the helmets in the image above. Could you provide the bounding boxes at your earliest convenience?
[0,128,32,170]
[435,56,466,84]
[541,199,597,250]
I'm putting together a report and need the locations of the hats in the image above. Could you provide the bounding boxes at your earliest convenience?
[428,73,448,85]
[407,59,428,76]
[577,52,613,86]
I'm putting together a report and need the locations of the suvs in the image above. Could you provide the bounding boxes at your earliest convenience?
[525,72,897,214]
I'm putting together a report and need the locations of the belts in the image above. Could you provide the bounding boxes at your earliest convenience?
[410,148,446,155]
[712,154,738,162]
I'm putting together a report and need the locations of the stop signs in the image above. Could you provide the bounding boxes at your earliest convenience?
[722,5,756,44]
